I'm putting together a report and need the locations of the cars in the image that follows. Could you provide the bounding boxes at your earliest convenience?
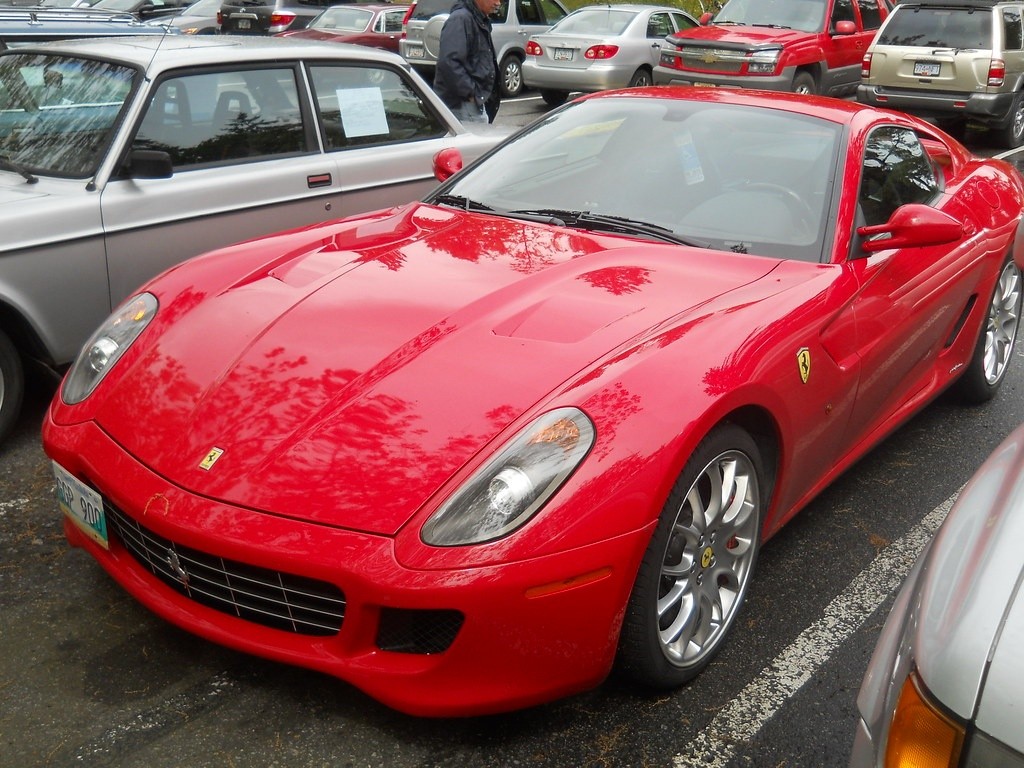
[855,0,1023,148]
[0,42,520,443]
[652,0,891,100]
[519,4,700,106]
[86,2,566,93]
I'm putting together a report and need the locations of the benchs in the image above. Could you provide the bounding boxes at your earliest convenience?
[272,105,415,149]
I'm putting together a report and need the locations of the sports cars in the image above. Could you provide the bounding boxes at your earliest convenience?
[41,89,1024,720]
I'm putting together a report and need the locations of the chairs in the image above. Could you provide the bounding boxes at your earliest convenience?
[611,22,626,33]
[573,20,591,34]
[585,110,690,223]
[204,92,252,160]
[150,79,193,167]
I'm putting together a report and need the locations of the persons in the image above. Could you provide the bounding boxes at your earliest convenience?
[434,0,502,125]
